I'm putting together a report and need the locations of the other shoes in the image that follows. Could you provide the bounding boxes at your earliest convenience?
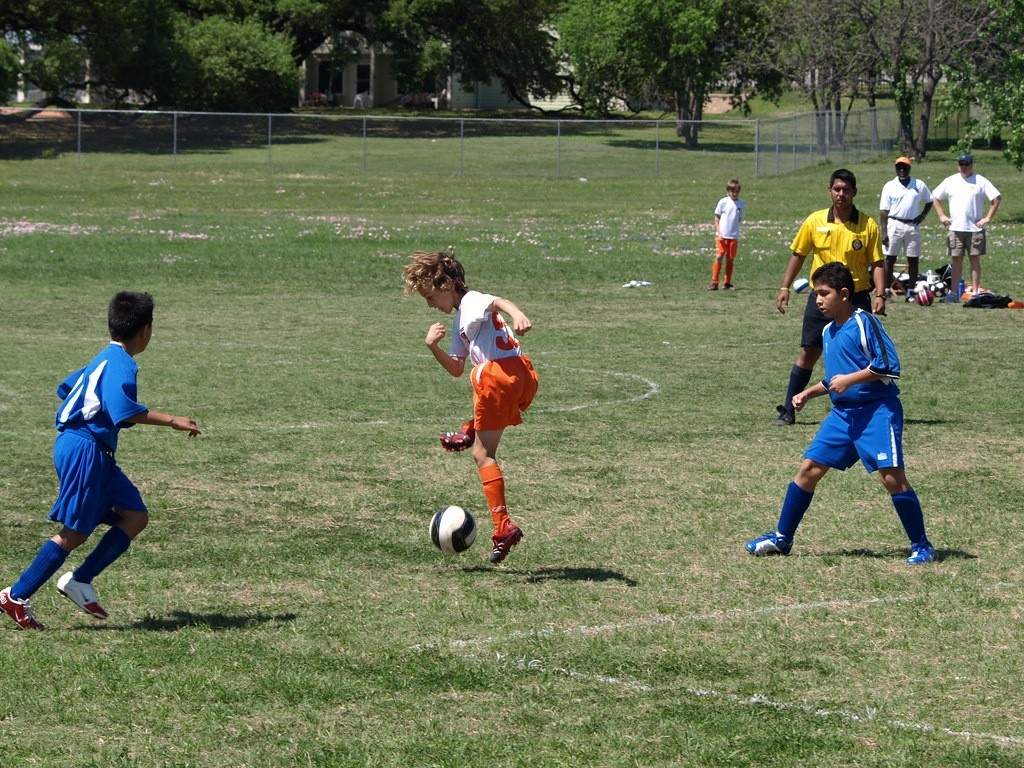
[939,292,959,303]
[707,283,718,290]
[905,291,915,302]
[723,283,736,289]
[883,290,891,302]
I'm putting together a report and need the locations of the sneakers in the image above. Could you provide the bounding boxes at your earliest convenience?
[490,521,524,564]
[772,405,795,425]
[57,571,108,619]
[745,529,794,555]
[0,586,45,631]
[906,542,938,565]
[438,429,475,451]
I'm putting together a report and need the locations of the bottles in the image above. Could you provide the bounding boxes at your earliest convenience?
[958,280,965,299]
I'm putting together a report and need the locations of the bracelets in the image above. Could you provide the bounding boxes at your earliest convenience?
[780,287,789,291]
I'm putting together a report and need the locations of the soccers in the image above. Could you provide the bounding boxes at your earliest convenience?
[917,290,933,306]
[931,281,949,297]
[793,278,810,293]
[429,504,478,554]
[916,281,930,293]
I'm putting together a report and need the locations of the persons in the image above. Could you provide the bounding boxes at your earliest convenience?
[708,179,745,290]
[403,249,539,564]
[879,156,933,303]
[0,292,202,631]
[773,168,886,426]
[932,154,1001,299]
[744,263,937,565]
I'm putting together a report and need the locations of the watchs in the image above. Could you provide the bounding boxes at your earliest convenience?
[877,293,887,300]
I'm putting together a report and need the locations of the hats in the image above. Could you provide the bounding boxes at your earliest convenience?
[895,156,911,166]
[956,155,973,163]
